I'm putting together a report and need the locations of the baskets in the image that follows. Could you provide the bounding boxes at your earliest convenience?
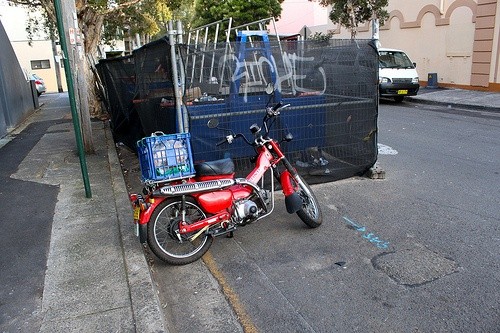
[136,132,197,181]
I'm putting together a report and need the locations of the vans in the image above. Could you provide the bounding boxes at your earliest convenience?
[379,48,419,103]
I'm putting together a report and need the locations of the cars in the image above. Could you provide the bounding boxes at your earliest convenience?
[28,73,46,96]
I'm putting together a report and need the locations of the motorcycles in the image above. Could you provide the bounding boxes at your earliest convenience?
[129,81,323,265]
